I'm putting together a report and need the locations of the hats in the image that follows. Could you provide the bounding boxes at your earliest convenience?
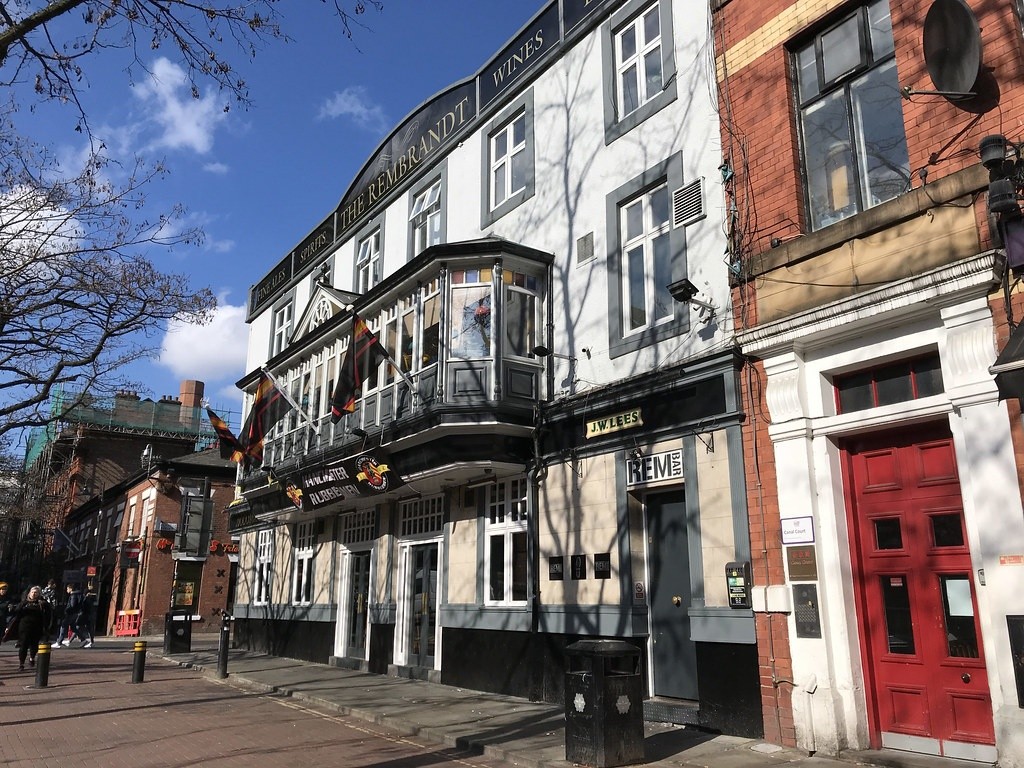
[0,582,8,588]
[85,589,92,593]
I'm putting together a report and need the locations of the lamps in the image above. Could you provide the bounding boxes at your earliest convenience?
[665,278,719,311]
[352,427,368,437]
[532,346,576,361]
[261,466,275,471]
[338,507,357,516]
[398,491,421,503]
[466,475,497,488]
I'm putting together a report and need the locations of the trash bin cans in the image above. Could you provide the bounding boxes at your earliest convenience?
[163,609,192,655]
[565,636,647,768]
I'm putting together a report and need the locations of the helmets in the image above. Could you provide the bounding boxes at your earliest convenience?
[476,305,491,315]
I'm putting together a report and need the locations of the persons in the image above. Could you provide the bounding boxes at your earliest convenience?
[40,579,58,642]
[4,586,51,672]
[0,581,14,647]
[51,584,91,648]
[22,585,34,601]
[61,586,98,646]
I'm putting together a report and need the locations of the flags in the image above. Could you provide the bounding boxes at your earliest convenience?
[206,408,247,463]
[236,371,296,467]
[330,312,390,425]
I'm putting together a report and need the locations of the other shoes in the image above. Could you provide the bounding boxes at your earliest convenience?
[61,640,71,646]
[29,658,35,667]
[51,642,61,649]
[84,643,93,648]
[79,640,87,648]
[18,666,24,671]
